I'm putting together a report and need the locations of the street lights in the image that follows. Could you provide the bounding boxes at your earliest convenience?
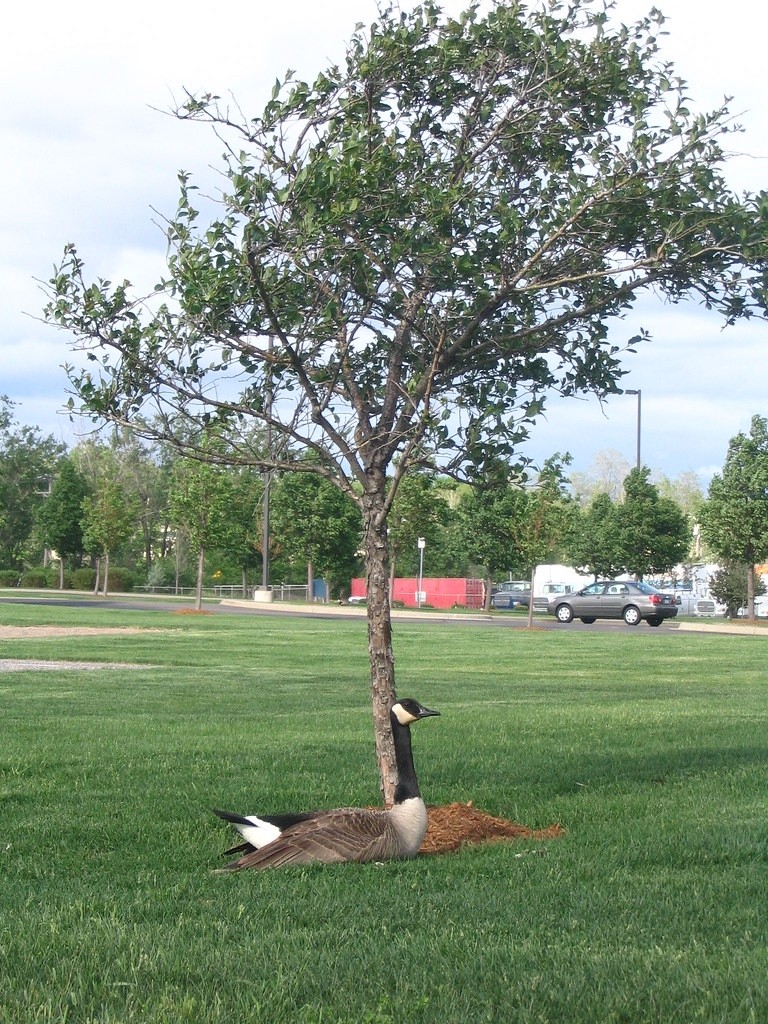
[625,389,641,468]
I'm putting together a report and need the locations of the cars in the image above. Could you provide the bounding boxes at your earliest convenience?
[547,581,678,627]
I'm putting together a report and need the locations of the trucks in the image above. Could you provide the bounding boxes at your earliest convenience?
[532,581,575,612]
[493,580,531,608]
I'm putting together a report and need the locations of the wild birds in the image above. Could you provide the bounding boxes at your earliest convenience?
[216,699,442,872]
